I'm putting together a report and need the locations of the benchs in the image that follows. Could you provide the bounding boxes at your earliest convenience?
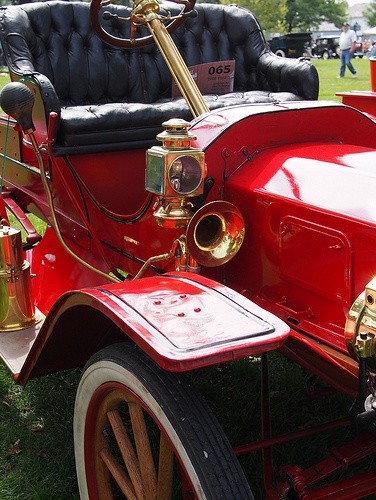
[0,0,320,156]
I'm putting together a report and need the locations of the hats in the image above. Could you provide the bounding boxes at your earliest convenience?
[342,22,350,27]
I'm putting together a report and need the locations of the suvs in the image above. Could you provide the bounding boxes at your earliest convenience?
[310,35,342,60]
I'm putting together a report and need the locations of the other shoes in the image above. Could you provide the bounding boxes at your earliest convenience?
[352,71,358,78]
[336,76,344,79]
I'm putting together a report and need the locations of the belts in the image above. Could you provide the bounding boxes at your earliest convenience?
[342,48,350,51]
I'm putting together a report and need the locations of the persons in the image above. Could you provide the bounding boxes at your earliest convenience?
[336,22,357,78]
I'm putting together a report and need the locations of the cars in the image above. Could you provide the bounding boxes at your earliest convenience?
[0,0,376,500]
[266,32,315,59]
